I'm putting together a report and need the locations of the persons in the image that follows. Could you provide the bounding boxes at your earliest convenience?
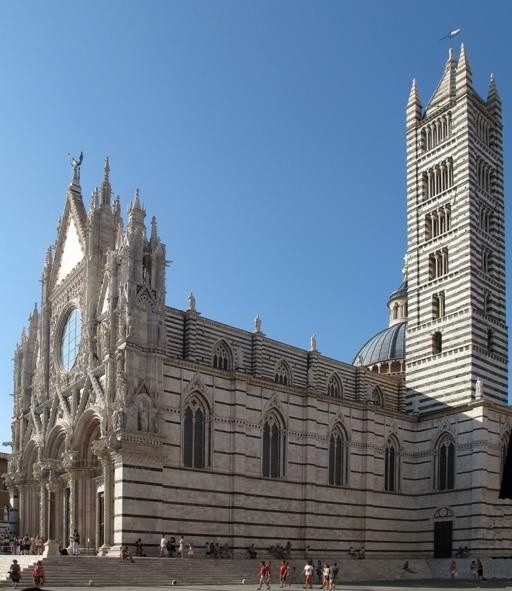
[348,547,365,559]
[456,546,468,559]
[33,561,44,588]
[8,559,21,589]
[120,528,338,591]
[0,529,81,556]
[448,561,457,578]
[470,559,483,586]
